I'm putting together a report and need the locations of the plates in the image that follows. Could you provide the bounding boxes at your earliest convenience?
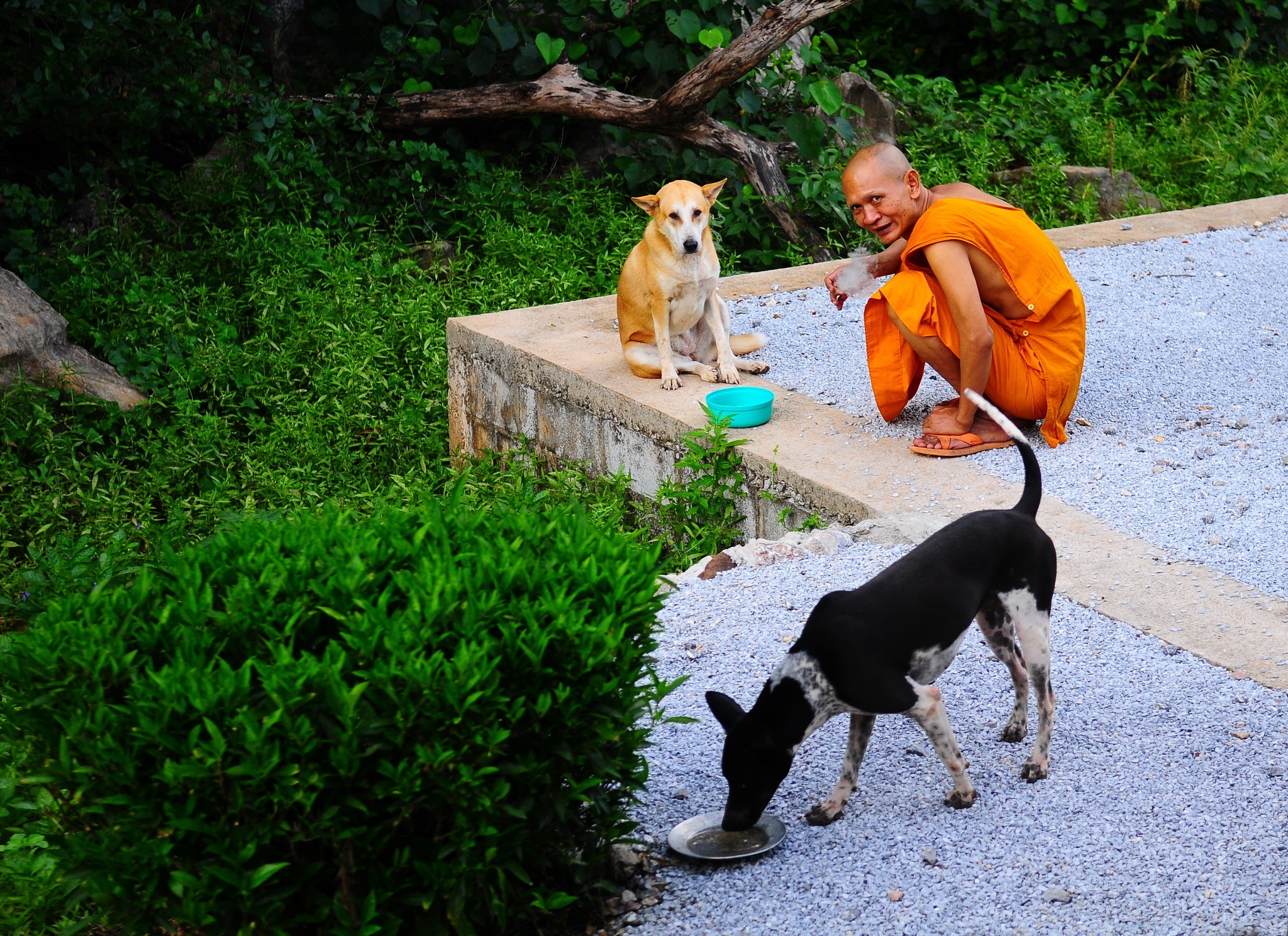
[668,810,787,859]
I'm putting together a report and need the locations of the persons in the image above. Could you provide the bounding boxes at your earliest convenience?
[824,144,1088,457]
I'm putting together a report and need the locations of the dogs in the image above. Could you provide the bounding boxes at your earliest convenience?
[703,387,1058,832]
[614,177,768,391]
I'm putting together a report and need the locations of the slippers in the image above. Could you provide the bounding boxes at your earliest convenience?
[910,433,1015,456]
[947,397,960,406]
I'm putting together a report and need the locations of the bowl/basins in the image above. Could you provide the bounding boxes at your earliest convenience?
[706,386,775,428]
